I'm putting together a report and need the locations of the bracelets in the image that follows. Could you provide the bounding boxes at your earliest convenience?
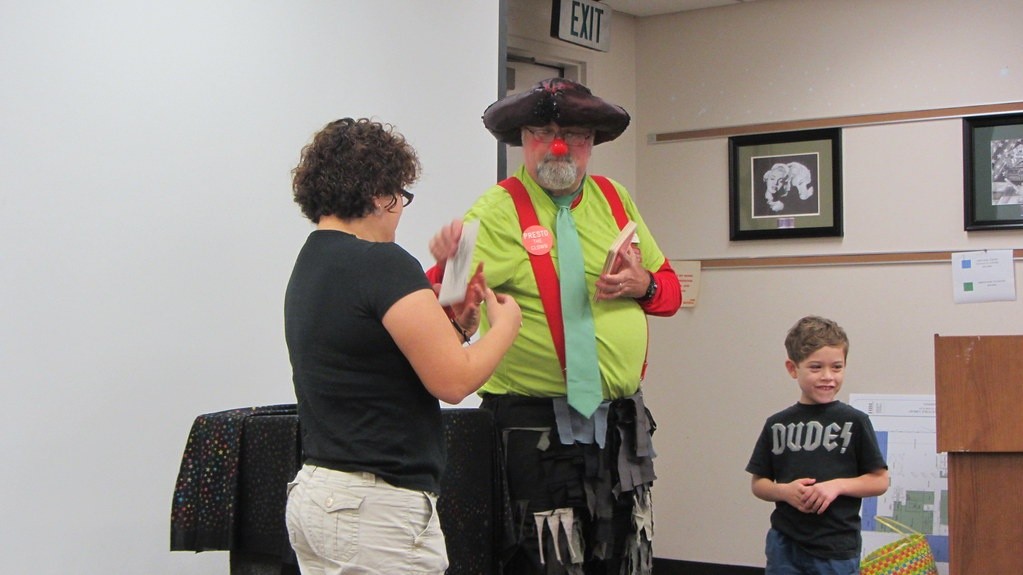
[451,319,470,342]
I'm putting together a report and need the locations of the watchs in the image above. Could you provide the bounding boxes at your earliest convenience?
[633,273,657,301]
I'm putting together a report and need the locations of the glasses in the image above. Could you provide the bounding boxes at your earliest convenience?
[524,126,592,146]
[397,187,414,207]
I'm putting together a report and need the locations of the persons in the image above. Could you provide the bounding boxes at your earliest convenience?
[754,161,818,215]
[284,117,522,575]
[745,316,889,575]
[424,77,681,575]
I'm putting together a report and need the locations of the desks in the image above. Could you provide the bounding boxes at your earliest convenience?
[170,405,518,575]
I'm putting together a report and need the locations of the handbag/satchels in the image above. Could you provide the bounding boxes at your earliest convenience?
[860,514,938,575]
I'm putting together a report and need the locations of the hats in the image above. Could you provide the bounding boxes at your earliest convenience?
[482,78,630,145]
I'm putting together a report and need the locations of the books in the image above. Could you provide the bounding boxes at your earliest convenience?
[593,220,638,302]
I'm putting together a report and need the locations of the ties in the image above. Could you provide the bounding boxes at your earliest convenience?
[540,171,604,420]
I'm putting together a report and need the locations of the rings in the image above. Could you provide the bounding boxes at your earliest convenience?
[619,283,622,290]
[476,300,484,305]
[520,322,523,327]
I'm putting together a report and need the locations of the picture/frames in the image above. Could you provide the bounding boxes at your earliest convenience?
[962,112,1023,231]
[727,128,845,241]
